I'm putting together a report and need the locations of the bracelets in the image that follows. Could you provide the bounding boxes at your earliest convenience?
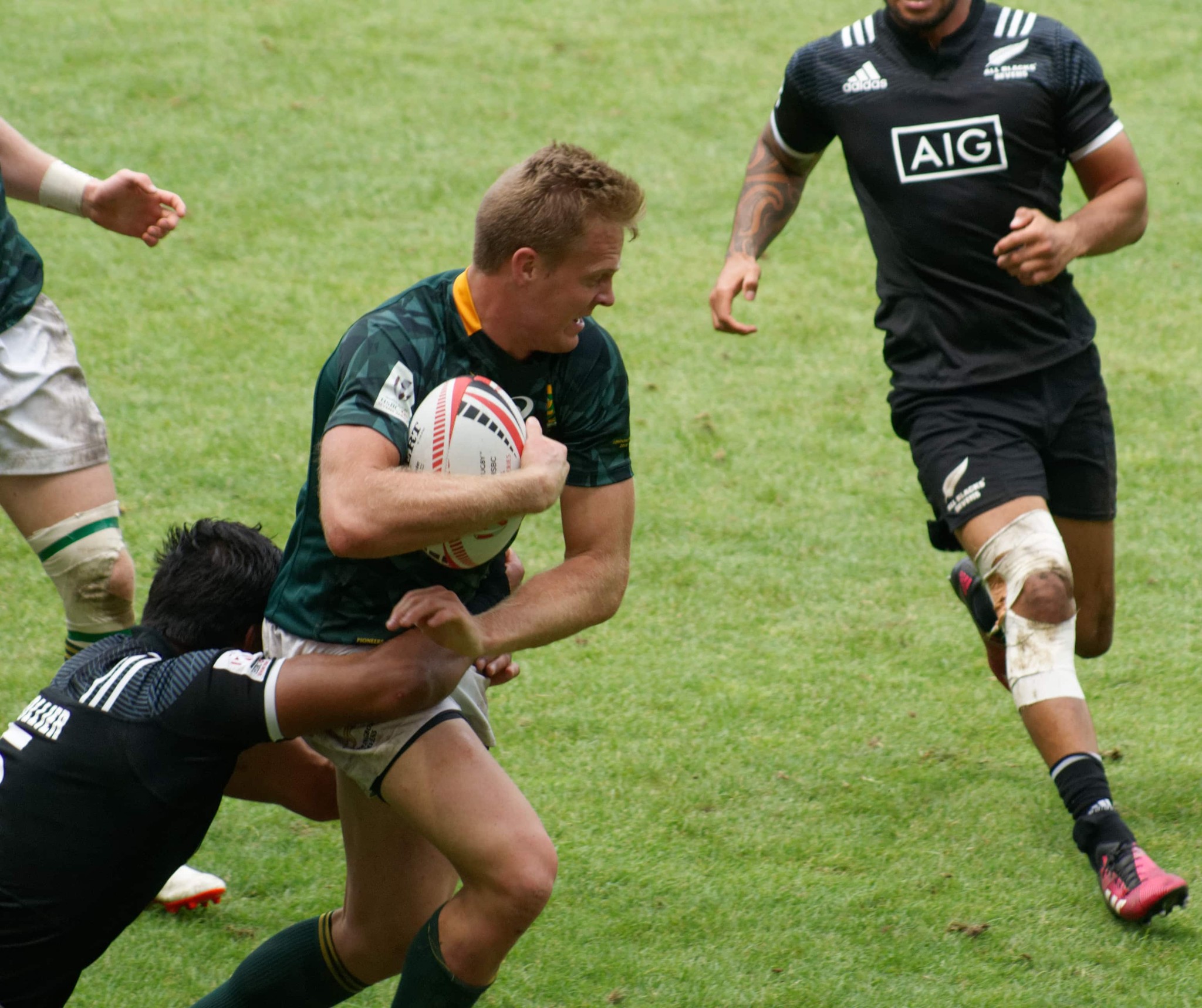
[39,159,90,218]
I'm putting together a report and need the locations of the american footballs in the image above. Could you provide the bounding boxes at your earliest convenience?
[403,373,528,570]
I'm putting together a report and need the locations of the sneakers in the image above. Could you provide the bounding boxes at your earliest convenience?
[154,864,225,912]
[947,556,1014,693]
[1094,836,1188,924]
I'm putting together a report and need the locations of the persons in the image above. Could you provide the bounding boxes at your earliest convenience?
[709,0,1191,923]
[194,140,647,1008]
[0,116,227,908]
[0,516,526,1008]
